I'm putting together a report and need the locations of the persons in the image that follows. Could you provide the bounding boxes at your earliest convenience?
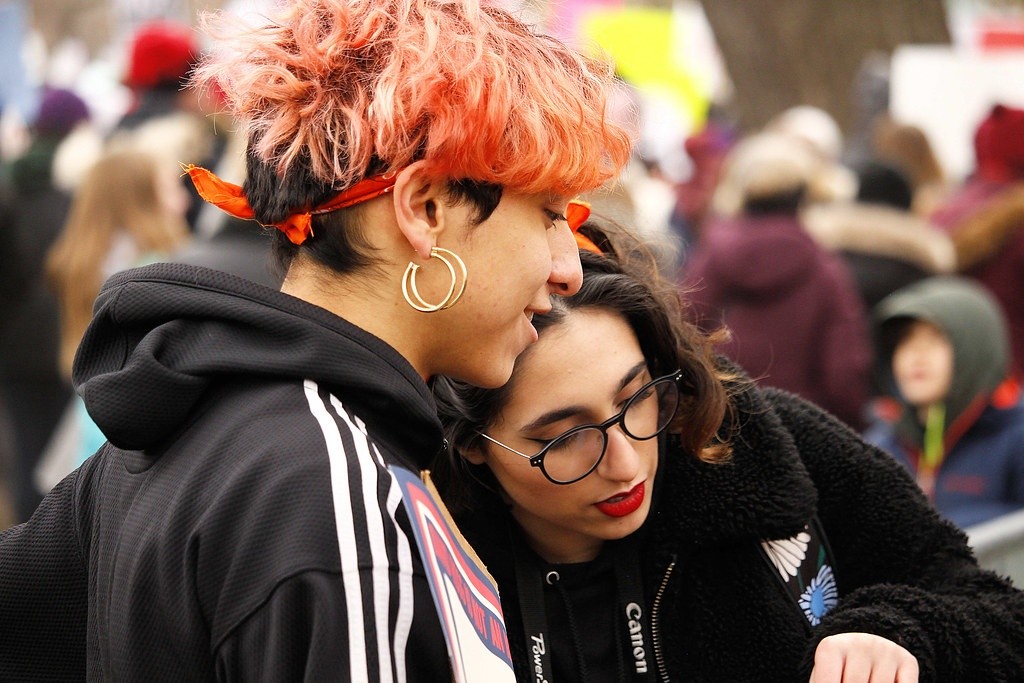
[2,25,1024,532]
[0,1,631,682]
[429,210,1022,683]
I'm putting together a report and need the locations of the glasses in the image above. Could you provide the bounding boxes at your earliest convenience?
[471,363,684,486]
